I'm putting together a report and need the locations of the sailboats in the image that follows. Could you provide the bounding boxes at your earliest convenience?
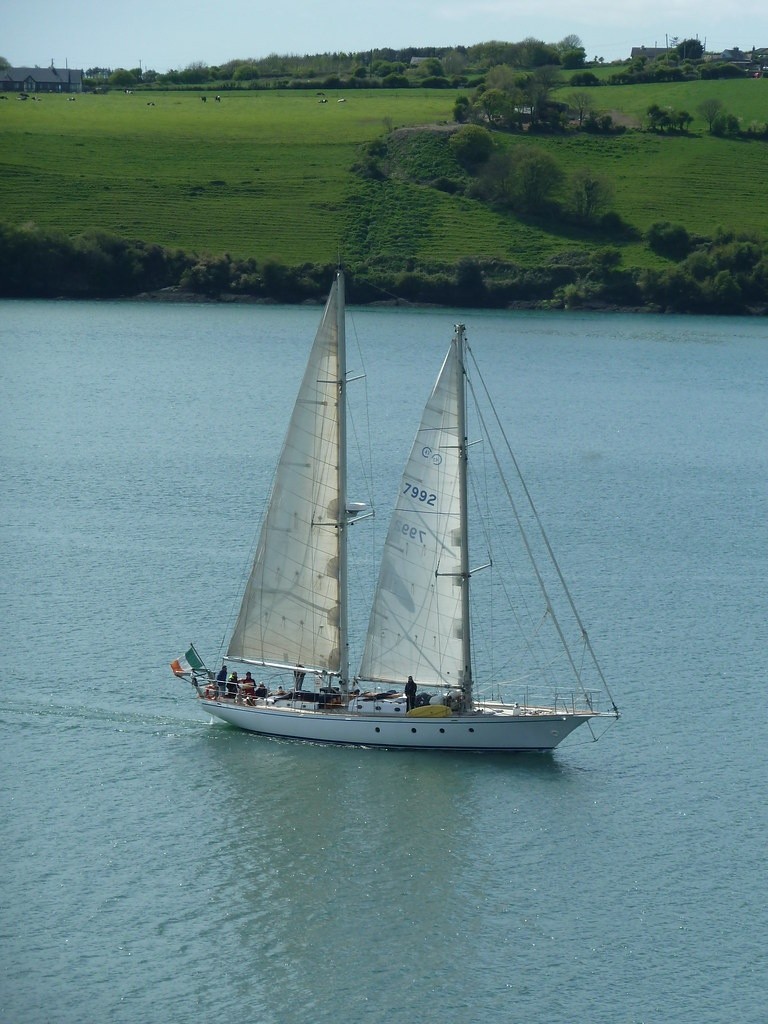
[175,271,622,750]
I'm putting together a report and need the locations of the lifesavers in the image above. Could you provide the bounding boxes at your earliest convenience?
[206,684,220,700]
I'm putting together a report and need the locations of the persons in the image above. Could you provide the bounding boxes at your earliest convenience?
[404,675,417,712]
[255,681,267,698]
[216,665,228,698]
[237,671,256,698]
[226,671,239,698]
